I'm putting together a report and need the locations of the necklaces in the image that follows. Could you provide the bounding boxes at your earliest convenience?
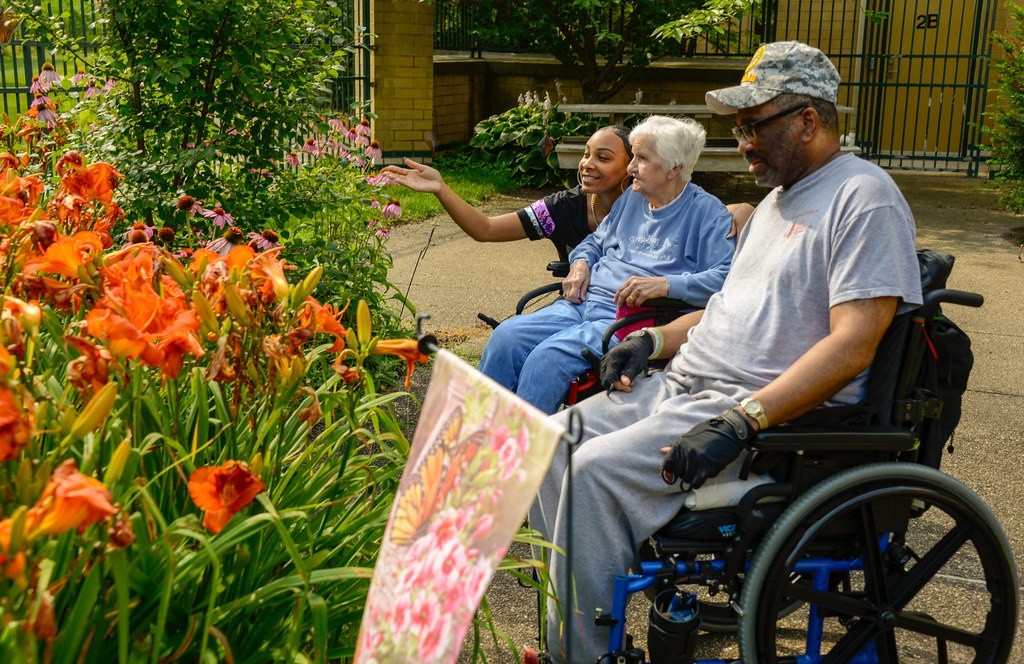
[591,193,600,226]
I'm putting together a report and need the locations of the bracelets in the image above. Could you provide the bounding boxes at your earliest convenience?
[640,327,663,360]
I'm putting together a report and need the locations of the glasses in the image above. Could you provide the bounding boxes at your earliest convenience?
[730,102,820,143]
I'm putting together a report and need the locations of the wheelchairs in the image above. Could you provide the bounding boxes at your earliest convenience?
[473,272,707,413]
[587,249,1019,664]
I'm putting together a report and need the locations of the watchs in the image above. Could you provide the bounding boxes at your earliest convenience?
[739,397,768,430]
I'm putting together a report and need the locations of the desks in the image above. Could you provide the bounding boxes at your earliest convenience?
[558,103,853,148]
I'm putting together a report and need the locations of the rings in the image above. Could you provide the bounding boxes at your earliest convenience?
[629,295,635,301]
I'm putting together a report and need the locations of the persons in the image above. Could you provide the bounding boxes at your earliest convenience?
[527,42,924,664]
[385,124,754,262]
[479,115,737,416]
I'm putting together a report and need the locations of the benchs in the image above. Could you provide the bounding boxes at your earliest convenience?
[557,137,858,172]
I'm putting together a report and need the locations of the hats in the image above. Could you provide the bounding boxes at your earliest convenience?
[705,41,841,116]
